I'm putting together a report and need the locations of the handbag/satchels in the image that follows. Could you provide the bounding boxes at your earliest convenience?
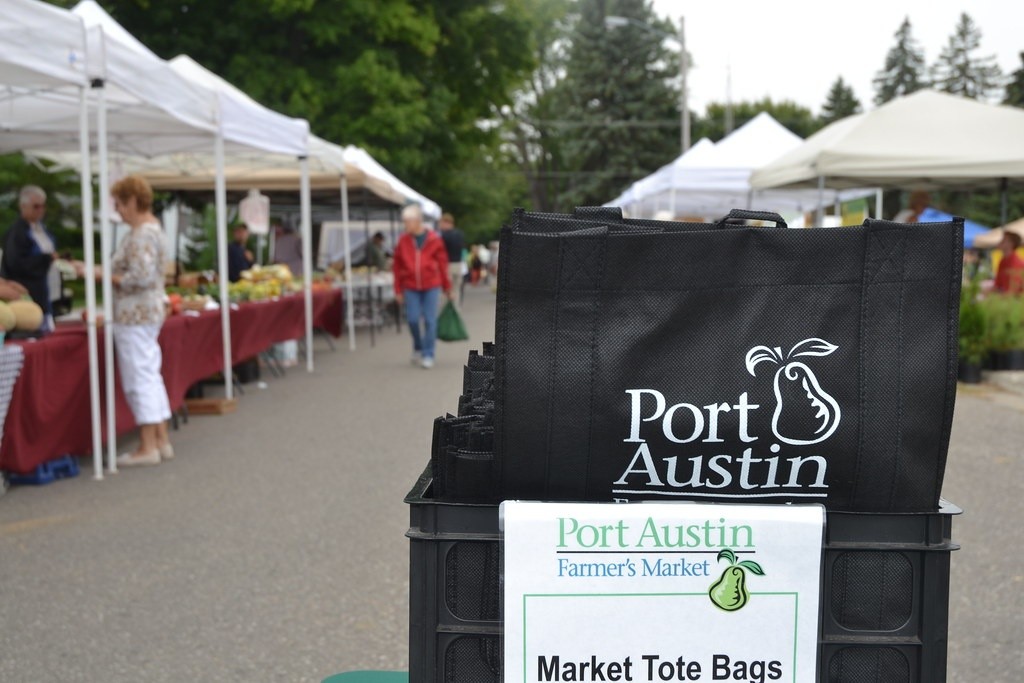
[430,206,963,683]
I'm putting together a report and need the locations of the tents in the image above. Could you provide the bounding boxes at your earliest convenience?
[128,136,440,353]
[43,1,232,479]
[26,52,316,374]
[601,83,1024,249]
[1,1,105,488]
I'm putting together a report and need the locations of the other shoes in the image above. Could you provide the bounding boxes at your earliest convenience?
[118,444,174,464]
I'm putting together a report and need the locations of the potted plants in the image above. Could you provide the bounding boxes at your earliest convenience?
[958,282,985,382]
[976,293,1024,371]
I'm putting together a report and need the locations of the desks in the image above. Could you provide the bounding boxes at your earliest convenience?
[1,279,344,475]
[336,263,467,328]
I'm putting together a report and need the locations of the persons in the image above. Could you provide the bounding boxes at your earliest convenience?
[3,186,59,324]
[102,176,175,470]
[992,230,1024,298]
[390,197,442,368]
[435,205,498,317]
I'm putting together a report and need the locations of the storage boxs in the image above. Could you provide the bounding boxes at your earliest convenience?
[403,459,960,682]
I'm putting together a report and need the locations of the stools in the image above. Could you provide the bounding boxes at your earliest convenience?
[9,454,78,480]
